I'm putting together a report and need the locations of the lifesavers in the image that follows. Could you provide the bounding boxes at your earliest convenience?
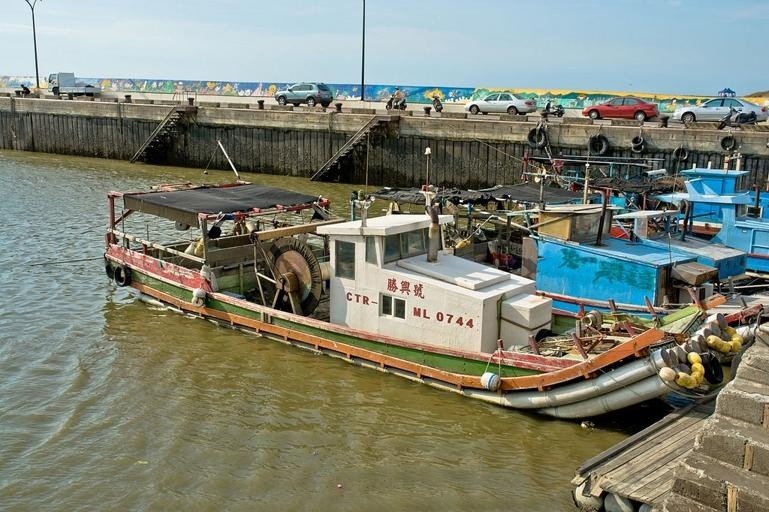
[673,145,689,161]
[721,135,736,152]
[631,135,644,153]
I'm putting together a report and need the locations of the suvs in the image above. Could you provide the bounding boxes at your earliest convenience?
[274,81,333,109]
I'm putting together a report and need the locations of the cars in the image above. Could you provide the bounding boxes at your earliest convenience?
[465,91,537,117]
[672,96,768,125]
[582,95,659,122]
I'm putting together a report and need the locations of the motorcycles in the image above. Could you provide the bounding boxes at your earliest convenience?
[433,96,444,113]
[717,101,758,131]
[385,94,407,110]
[542,99,565,117]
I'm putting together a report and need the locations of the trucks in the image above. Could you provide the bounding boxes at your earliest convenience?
[43,72,99,98]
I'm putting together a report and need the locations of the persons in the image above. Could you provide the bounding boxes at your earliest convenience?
[390,86,402,109]
[195,226,221,257]
[19,84,30,98]
